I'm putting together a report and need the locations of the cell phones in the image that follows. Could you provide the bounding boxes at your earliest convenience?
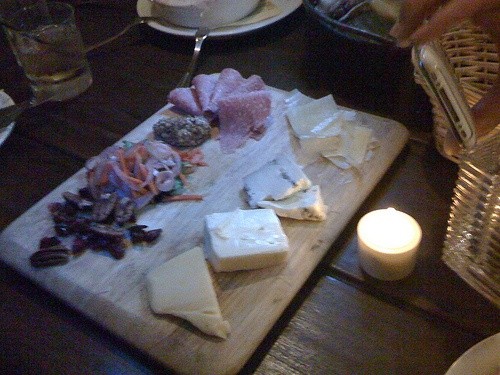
[418,40,477,152]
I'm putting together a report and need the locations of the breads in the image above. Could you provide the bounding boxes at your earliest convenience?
[146,246,231,339]
[203,208,288,272]
[242,153,327,221]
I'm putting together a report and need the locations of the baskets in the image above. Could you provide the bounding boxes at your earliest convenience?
[410,17,500,164]
[441,124,500,309]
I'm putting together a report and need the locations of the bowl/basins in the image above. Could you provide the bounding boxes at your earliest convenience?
[357,208,422,282]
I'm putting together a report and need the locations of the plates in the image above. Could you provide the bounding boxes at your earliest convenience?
[136,0,304,37]
[444,331,500,375]
[0,86,17,145]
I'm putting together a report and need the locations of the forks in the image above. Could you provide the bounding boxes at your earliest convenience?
[176,27,211,88]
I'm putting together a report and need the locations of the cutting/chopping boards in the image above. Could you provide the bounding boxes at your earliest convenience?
[0,72,407,375]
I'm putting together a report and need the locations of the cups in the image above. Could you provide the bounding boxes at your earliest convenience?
[437,133,500,308]
[0,2,93,101]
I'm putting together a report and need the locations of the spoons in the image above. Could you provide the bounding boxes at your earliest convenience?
[77,15,159,56]
